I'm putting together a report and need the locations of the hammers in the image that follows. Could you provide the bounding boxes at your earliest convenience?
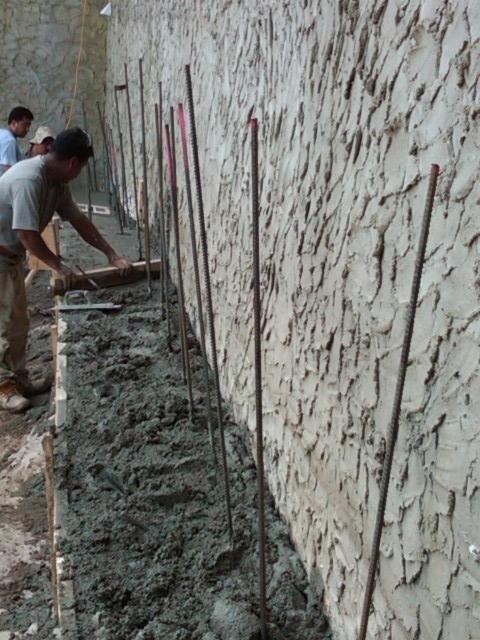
[75,264,107,298]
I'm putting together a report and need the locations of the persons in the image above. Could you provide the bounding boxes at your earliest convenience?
[0,107,34,175]
[22,126,56,160]
[1,126,134,414]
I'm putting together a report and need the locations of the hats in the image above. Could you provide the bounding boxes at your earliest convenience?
[28,125,57,145]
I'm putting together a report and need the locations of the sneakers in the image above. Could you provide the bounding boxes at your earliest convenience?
[0,376,48,413]
